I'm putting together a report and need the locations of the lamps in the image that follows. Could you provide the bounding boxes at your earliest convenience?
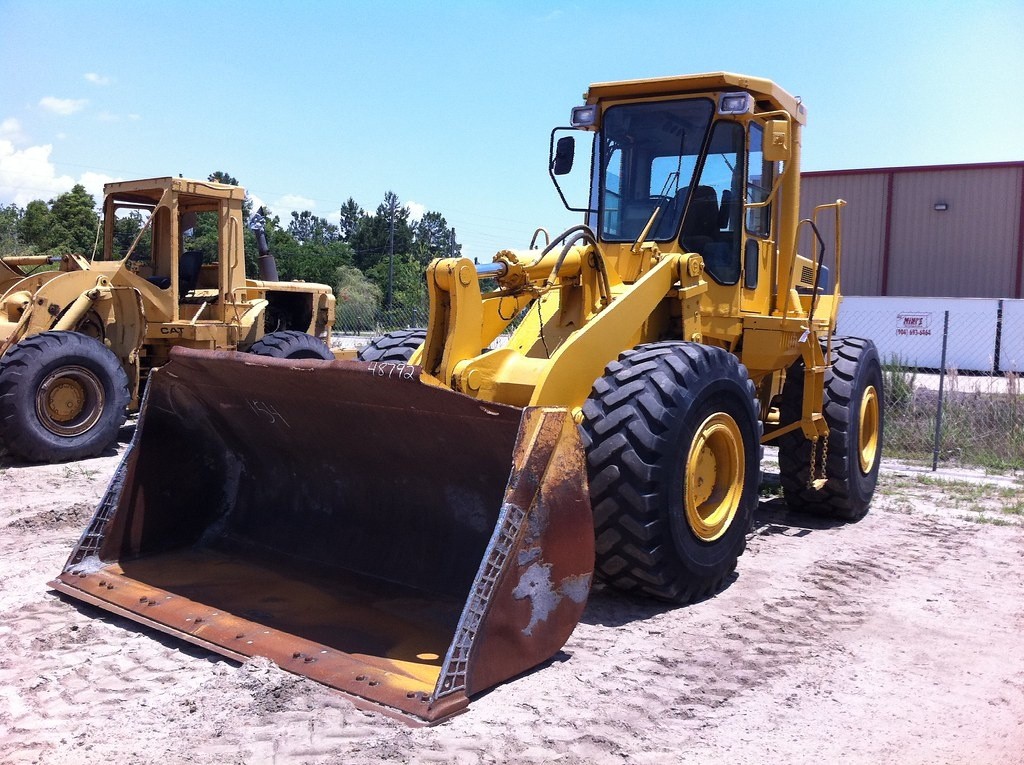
[933,203,949,211]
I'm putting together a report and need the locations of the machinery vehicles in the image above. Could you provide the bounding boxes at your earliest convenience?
[0,172,357,463]
[44,70,889,736]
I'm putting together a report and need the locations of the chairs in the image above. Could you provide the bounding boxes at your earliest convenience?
[148,251,203,298]
[671,185,720,252]
